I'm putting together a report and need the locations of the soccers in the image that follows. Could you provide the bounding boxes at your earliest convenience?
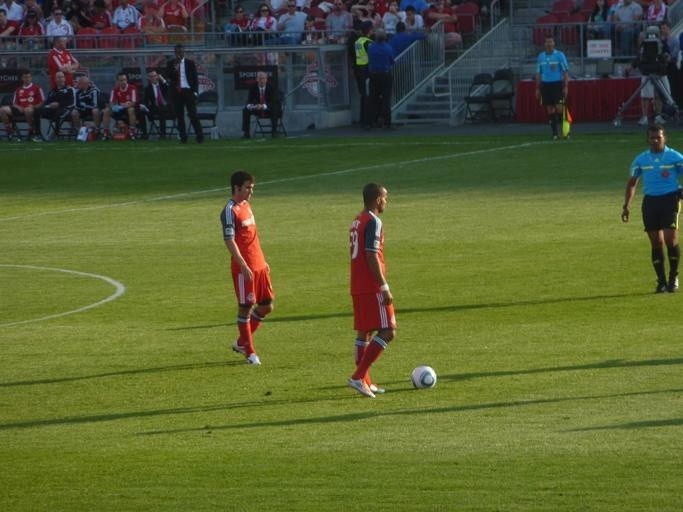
[411,365,437,389]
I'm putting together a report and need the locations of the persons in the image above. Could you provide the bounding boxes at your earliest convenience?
[620,123,683,292]
[535,1,683,141]
[349,182,398,399]
[220,169,275,365]
[1,1,208,143]
[227,0,509,143]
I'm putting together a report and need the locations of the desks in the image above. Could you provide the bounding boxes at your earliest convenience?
[510,73,661,126]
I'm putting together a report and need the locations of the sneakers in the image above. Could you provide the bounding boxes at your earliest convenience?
[656,277,666,293]
[667,274,679,292]
[231,341,247,356]
[369,384,386,394]
[246,354,262,365]
[653,114,666,124]
[6,133,135,143]
[348,376,375,399]
[637,116,647,125]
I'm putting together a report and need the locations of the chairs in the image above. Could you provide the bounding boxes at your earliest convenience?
[460,73,494,125]
[0,1,648,52]
[487,65,518,121]
[1,71,288,142]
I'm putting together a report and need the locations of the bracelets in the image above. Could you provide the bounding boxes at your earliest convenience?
[622,205,632,209]
[380,283,390,292]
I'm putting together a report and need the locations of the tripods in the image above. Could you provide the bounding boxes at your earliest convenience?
[613,77,683,130]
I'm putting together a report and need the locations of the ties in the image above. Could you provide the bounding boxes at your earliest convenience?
[157,85,164,106]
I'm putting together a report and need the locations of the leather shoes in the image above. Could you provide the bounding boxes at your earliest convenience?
[141,134,148,140]
[240,132,251,139]
[159,133,166,141]
